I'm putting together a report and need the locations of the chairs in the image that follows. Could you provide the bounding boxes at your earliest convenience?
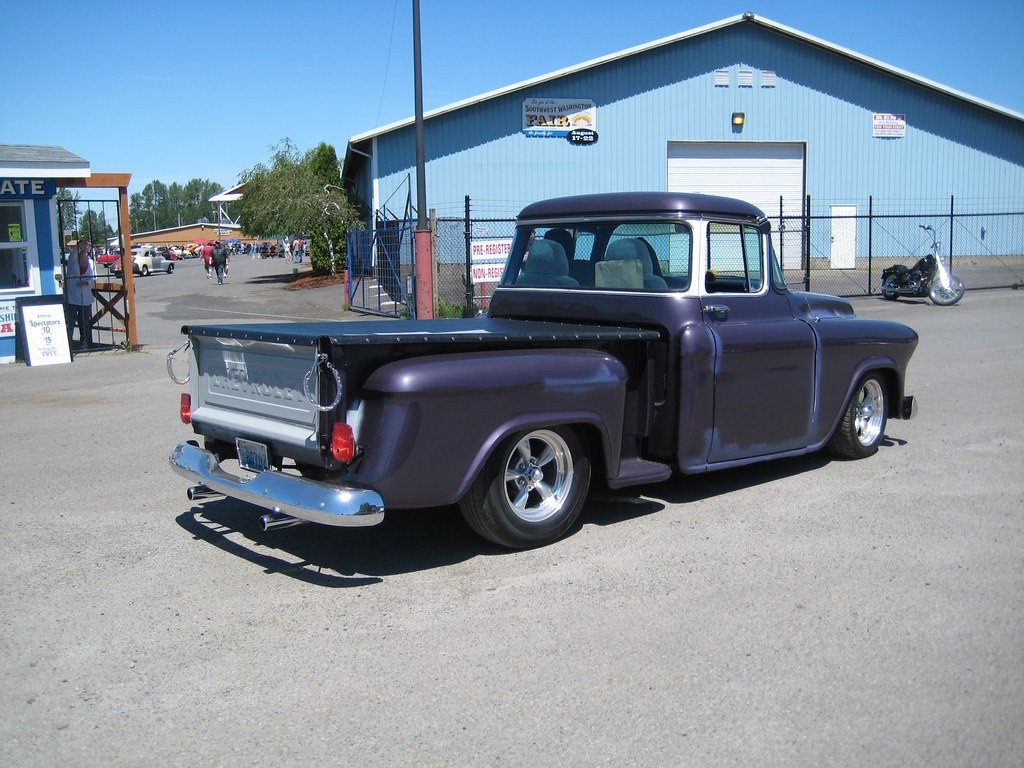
[516,239,580,290]
[606,238,668,290]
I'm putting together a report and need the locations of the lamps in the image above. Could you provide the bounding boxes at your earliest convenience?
[732,112,744,125]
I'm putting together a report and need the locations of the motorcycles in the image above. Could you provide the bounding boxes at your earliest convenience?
[880,224,965,306]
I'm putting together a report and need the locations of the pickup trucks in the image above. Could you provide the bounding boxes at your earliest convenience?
[165,190,921,552]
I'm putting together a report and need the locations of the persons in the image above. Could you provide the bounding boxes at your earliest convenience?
[520,228,670,290]
[230,236,307,264]
[199,241,231,285]
[88,244,207,261]
[64,238,95,351]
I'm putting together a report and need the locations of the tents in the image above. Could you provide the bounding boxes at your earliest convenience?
[224,238,240,243]
[194,237,210,245]
[110,239,119,246]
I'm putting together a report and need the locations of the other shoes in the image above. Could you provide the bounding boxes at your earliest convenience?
[209,275,212,280]
[223,273,228,278]
[217,281,221,285]
[207,274,209,279]
[78,342,93,350]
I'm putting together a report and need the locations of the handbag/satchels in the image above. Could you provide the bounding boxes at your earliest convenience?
[209,256,213,265]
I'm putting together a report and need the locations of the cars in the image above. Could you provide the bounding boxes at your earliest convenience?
[96,243,200,278]
